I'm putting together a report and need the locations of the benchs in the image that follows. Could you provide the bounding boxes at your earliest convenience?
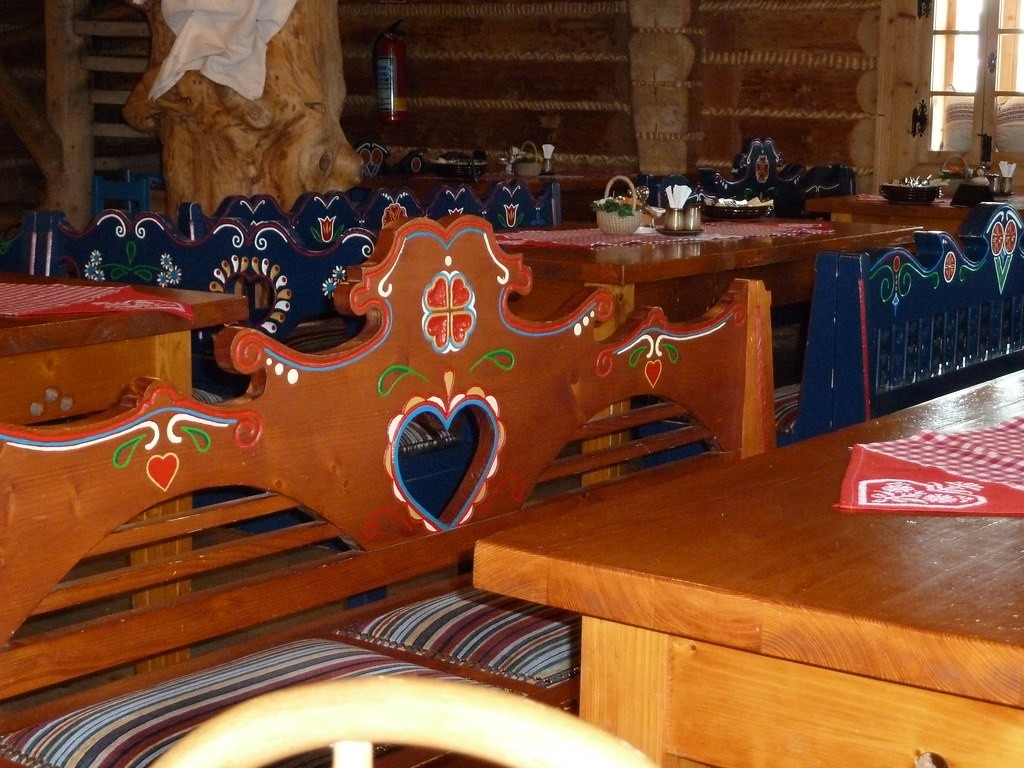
[625,135,858,223]
[18,169,569,401]
[705,202,1023,446]
[0,213,777,768]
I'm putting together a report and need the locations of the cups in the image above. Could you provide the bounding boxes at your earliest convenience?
[1000,177,1013,196]
[987,174,1001,194]
[664,208,702,231]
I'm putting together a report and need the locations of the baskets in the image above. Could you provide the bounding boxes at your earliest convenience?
[938,154,970,197]
[594,174,642,237]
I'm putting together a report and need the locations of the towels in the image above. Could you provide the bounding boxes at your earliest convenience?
[147,0,299,101]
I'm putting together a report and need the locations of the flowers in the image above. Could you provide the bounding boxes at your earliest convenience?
[591,186,658,217]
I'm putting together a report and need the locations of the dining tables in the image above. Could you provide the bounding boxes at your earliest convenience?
[804,186,1024,262]
[2,273,251,679]
[491,216,926,393]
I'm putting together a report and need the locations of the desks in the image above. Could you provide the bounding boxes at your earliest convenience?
[468,367,1024,767]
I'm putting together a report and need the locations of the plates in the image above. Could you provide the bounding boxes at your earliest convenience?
[435,163,487,179]
[655,228,704,235]
[879,184,943,202]
[698,206,773,219]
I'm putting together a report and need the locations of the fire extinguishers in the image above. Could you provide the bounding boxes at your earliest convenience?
[371,18,410,124]
